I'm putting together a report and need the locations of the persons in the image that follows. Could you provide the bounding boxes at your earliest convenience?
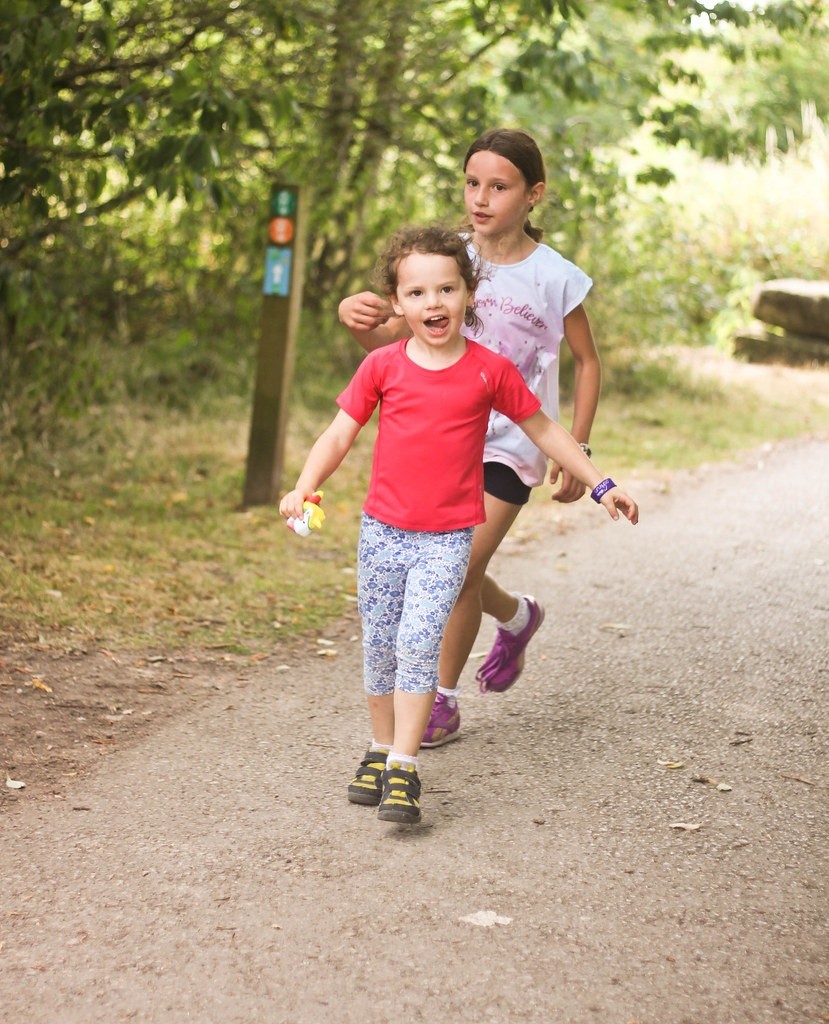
[336,129,602,749]
[279,229,638,823]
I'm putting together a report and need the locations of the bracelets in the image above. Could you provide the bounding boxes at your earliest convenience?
[589,476,617,504]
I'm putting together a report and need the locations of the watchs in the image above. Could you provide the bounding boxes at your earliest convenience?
[576,443,592,458]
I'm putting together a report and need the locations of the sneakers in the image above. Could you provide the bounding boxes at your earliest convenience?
[377,757,422,823]
[347,747,389,805]
[420,691,461,747]
[476,596,545,694]
